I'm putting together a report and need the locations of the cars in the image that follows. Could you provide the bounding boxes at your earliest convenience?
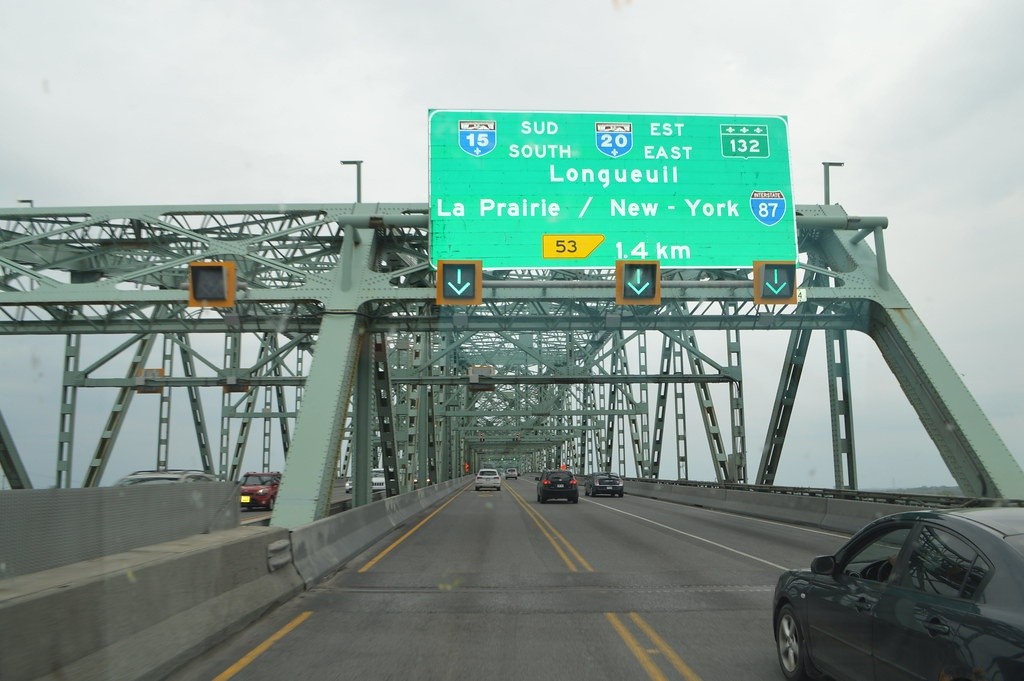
[772,505,1024,681]
[345,476,353,492]
[584,472,624,497]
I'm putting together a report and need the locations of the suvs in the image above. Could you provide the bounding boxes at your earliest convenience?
[372,468,386,491]
[474,469,502,491]
[114,469,219,485]
[236,471,282,511]
[535,470,579,504]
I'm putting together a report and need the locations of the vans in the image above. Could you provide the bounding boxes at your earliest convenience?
[505,468,517,479]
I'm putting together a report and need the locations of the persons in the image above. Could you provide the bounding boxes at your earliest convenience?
[888,553,897,565]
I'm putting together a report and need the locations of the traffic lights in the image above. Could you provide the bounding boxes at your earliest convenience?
[437,260,484,305]
[753,260,798,305]
[188,260,235,308]
[616,261,661,306]
